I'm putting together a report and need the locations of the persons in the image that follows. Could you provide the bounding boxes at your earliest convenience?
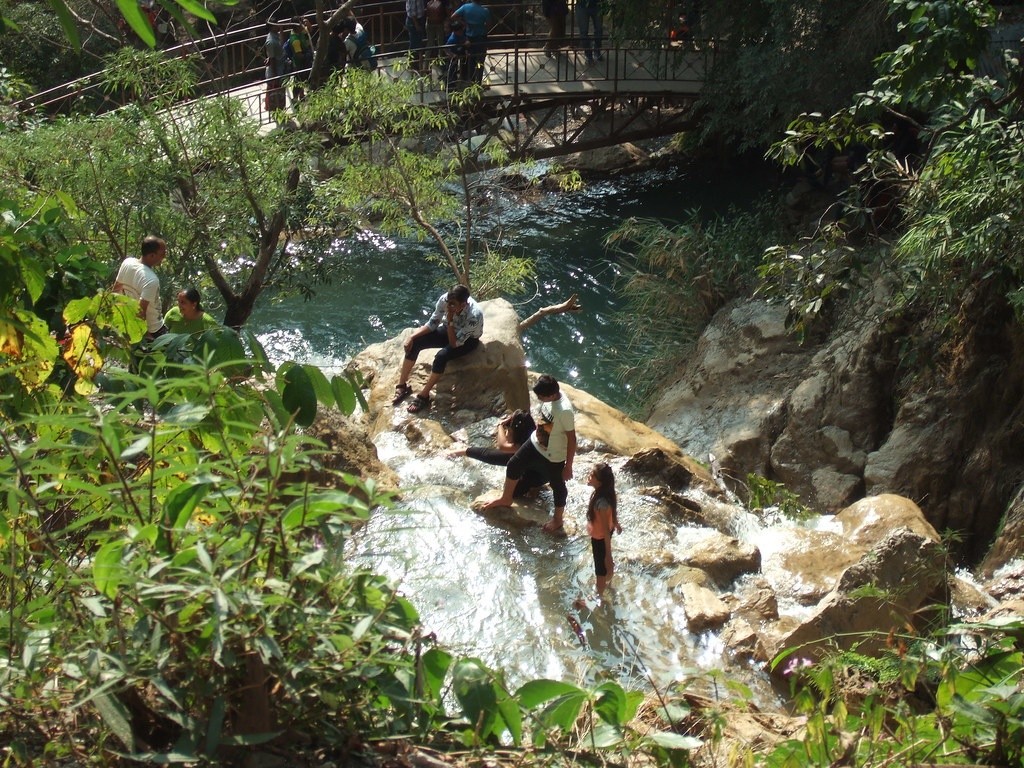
[482,373,578,531]
[261,0,491,112]
[445,408,549,498]
[112,238,170,343]
[392,285,484,413]
[164,289,219,334]
[542,0,604,65]
[585,461,623,602]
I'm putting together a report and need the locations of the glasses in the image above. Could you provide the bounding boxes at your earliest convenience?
[445,300,458,307]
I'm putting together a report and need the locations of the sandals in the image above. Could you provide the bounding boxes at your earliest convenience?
[393,384,413,404]
[407,392,432,413]
